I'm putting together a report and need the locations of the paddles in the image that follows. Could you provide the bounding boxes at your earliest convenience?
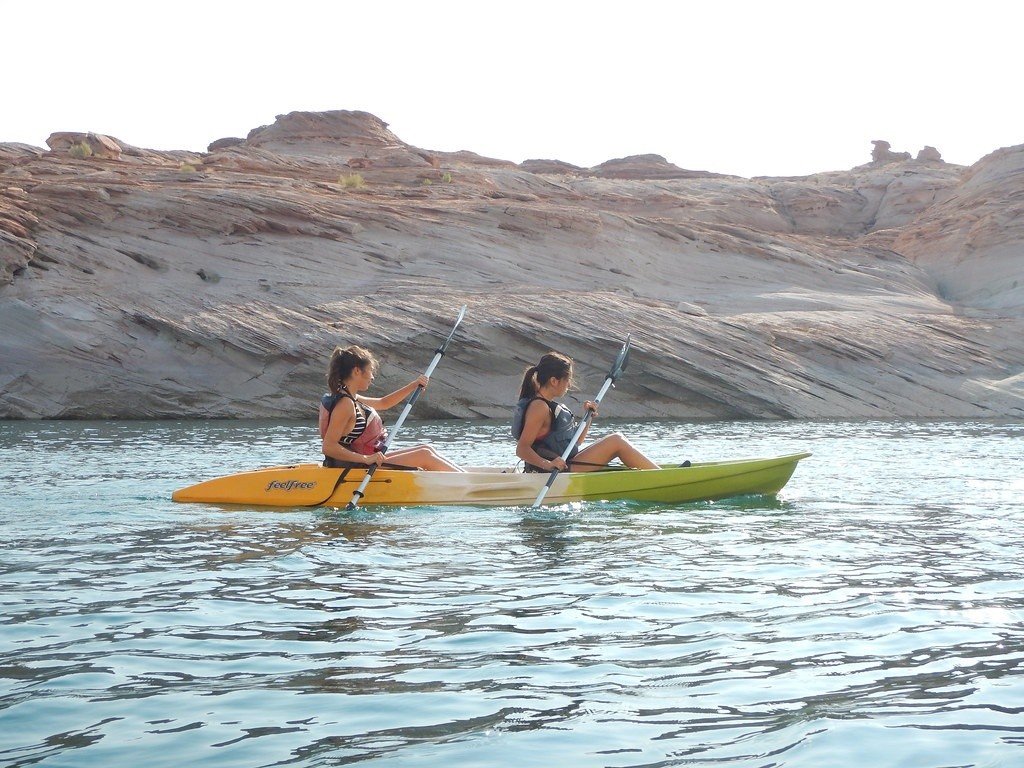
[532,331,632,509]
[344,303,469,510]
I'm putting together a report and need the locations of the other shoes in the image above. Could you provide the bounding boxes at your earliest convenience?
[680,461,691,467]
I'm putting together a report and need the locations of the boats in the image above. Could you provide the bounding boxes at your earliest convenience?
[169,450,815,511]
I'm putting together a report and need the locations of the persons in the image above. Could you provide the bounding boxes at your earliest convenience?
[319,346,467,472]
[511,352,691,472]
